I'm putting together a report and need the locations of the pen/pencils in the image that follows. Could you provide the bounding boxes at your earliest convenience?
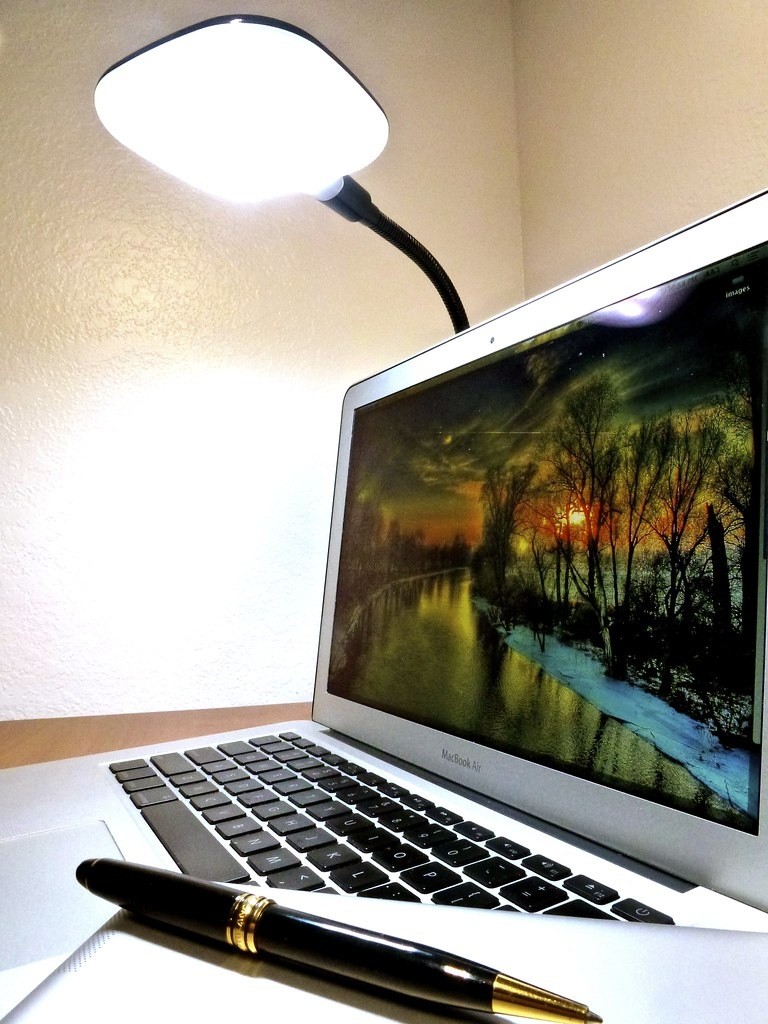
[73,855,605,1024]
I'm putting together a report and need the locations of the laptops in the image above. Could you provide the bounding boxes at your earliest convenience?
[0,189,768,1024]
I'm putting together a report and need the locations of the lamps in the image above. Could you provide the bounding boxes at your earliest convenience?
[93,16,470,334]
